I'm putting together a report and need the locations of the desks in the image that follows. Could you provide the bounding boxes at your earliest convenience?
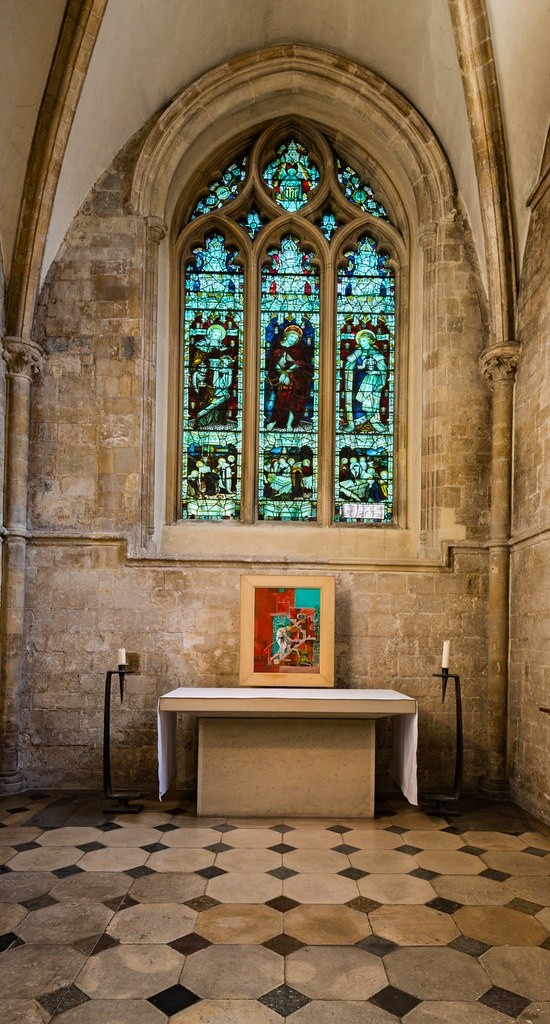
[157,687,423,821]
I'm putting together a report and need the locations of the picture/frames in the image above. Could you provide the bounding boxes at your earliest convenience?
[239,573,336,688]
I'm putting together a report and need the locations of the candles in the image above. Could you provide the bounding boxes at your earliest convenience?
[442,637,451,671]
[118,645,126,666]
